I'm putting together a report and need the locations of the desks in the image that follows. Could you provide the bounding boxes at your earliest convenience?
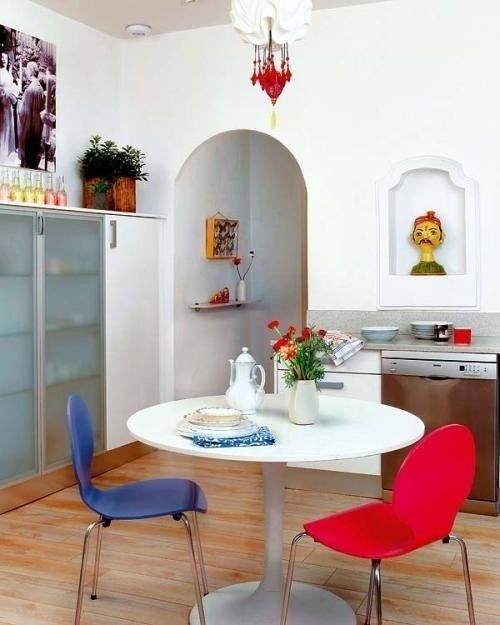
[125,393,426,624]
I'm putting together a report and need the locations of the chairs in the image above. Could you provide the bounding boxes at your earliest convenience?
[64,393,215,624]
[280,422,483,624]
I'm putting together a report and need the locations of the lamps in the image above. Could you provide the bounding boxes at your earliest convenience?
[229,0,313,130]
[125,23,152,38]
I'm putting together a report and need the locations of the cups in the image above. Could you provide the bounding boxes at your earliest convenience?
[453,327,471,345]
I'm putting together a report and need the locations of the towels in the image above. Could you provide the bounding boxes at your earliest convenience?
[193,428,277,448]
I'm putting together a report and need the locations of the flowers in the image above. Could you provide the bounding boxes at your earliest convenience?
[264,310,341,380]
[232,249,254,280]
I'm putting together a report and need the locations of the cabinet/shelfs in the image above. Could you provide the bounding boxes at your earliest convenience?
[265,347,384,498]
[1,200,169,514]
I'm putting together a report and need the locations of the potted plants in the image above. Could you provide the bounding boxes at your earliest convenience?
[78,135,148,212]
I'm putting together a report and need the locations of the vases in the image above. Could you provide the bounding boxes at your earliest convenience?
[236,280,246,301]
[287,381,319,424]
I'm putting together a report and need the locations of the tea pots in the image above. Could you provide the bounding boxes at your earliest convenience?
[225,347,266,415]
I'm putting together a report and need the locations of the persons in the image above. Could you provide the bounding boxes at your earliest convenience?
[408,211,448,274]
[0,43,55,173]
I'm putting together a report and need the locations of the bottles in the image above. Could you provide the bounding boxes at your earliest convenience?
[57,175,67,206]
[24,171,35,203]
[11,169,24,202]
[209,288,229,304]
[35,172,45,205]
[0,167,11,201]
[45,173,57,205]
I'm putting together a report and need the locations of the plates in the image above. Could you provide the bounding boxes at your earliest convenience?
[176,407,260,439]
[410,321,454,340]
[361,326,399,340]
